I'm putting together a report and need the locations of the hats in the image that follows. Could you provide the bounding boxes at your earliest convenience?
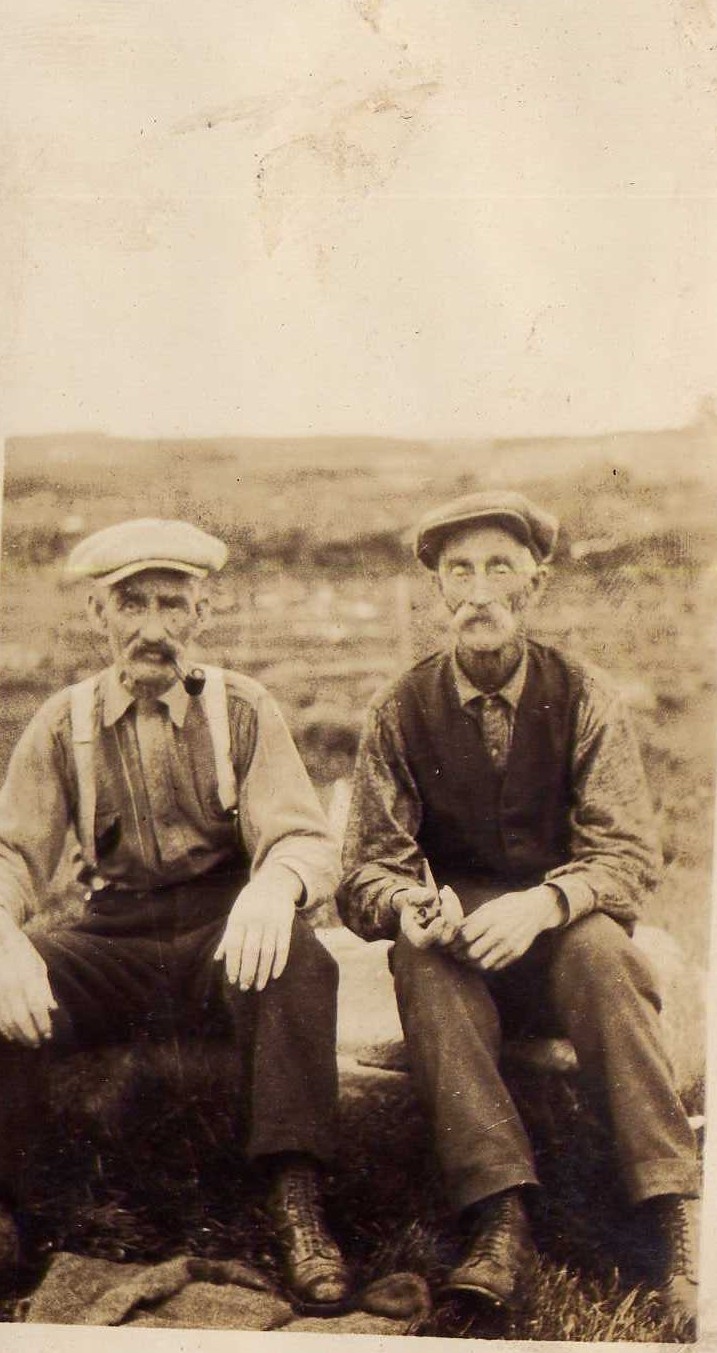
[67,518,230,586]
[413,493,562,570]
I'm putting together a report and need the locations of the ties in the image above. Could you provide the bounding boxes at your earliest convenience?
[480,696,513,776]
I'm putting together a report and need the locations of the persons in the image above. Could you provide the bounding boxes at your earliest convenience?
[0,517,342,1314]
[334,491,700,1319]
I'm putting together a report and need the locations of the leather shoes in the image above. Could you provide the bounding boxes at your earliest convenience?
[649,1198,698,1332]
[446,1196,539,1305]
[264,1163,355,1316]
